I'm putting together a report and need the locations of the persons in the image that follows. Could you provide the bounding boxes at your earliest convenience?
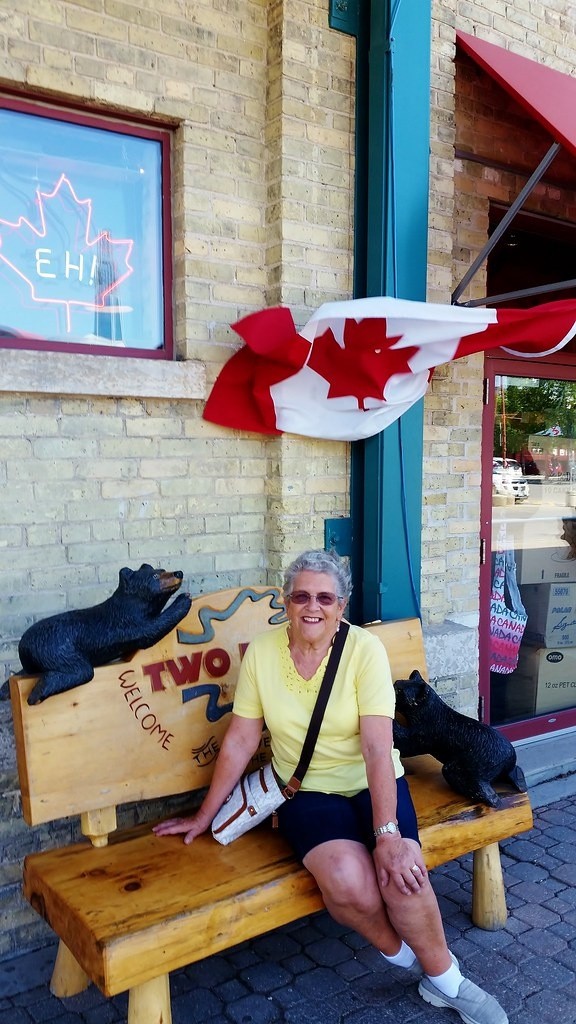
[151,548,509,1024]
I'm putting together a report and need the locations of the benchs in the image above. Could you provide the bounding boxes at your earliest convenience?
[0,586,533,1024]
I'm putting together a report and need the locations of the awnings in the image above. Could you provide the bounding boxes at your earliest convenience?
[450,29,576,306]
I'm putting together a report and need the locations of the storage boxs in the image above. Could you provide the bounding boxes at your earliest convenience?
[505,505,576,586]
[502,582,576,648]
[500,647,576,722]
[502,434,576,484]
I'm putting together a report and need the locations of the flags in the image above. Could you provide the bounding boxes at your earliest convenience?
[199,291,576,442]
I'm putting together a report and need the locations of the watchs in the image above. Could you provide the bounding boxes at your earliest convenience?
[373,821,399,836]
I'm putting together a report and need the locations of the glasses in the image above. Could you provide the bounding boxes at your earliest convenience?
[287,589,344,606]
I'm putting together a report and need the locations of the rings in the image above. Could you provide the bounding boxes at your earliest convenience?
[411,864,420,871]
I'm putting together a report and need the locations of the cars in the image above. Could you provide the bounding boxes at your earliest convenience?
[493,457,529,502]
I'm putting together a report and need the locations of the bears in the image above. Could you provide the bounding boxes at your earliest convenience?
[392,670,527,809]
[0,563,193,706]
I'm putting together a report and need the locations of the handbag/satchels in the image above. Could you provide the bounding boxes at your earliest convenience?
[487,509,528,674]
[211,761,301,847]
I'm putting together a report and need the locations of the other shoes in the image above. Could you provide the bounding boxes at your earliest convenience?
[406,948,460,981]
[418,973,509,1024]
